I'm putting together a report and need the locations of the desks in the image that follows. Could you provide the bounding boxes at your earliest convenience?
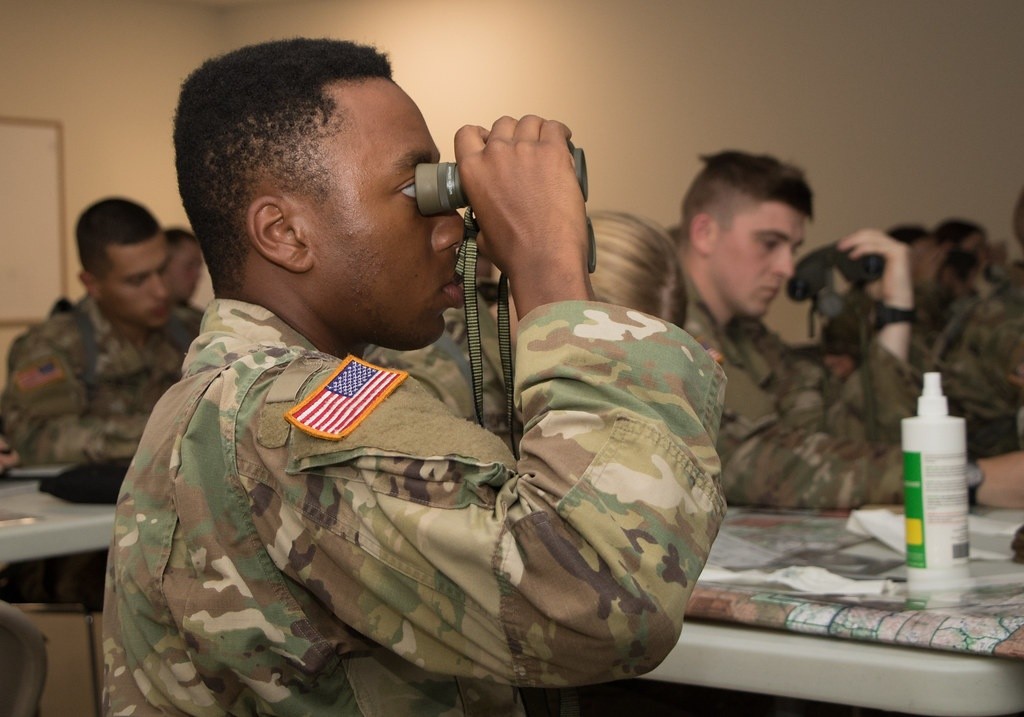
[633,506,1024,717]
[0,482,117,566]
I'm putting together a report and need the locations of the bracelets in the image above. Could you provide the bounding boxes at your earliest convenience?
[869,300,916,330]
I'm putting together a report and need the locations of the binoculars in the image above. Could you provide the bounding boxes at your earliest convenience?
[784,241,886,318]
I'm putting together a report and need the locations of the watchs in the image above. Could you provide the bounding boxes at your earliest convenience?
[967,455,983,505]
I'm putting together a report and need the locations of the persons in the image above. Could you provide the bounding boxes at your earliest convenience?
[357,209,689,460]
[1,197,208,506]
[671,148,1024,515]
[823,188,1024,461]
[162,228,203,303]
[102,37,728,717]
